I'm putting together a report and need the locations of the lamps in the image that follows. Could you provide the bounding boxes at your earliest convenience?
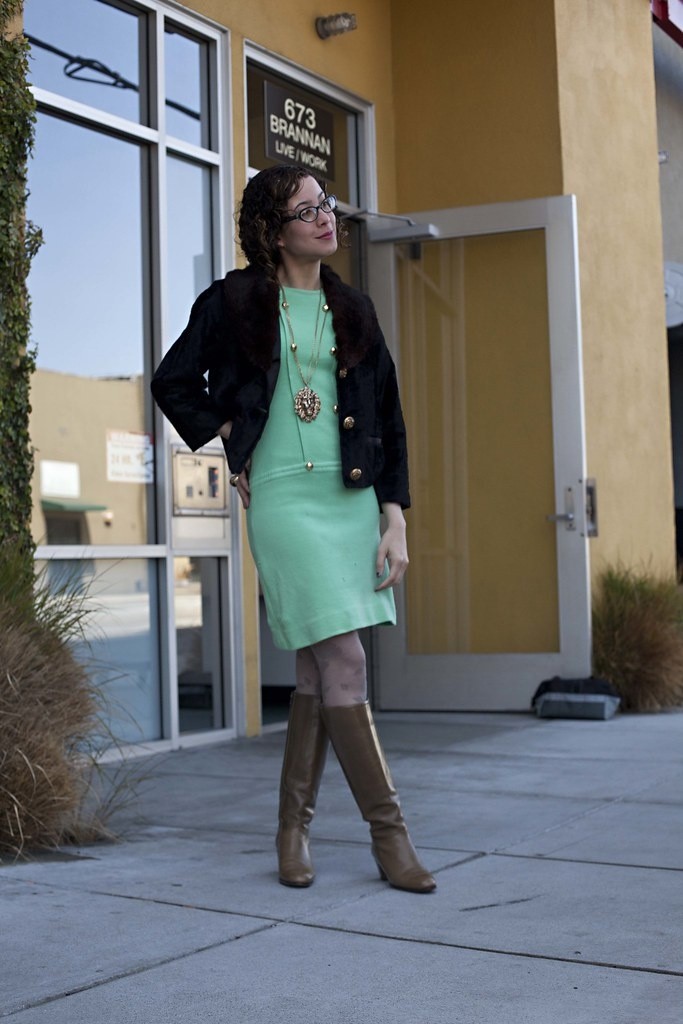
[315,13,358,39]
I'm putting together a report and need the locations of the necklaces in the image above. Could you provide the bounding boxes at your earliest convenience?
[280,281,332,423]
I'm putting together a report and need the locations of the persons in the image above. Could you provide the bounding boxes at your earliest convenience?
[148,167,443,894]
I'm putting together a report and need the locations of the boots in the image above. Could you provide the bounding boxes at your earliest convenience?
[319,700,437,893]
[276,689,332,887]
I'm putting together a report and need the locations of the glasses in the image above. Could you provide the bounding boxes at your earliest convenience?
[281,194,337,223]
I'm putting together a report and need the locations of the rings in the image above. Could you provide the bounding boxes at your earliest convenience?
[230,476,240,487]
